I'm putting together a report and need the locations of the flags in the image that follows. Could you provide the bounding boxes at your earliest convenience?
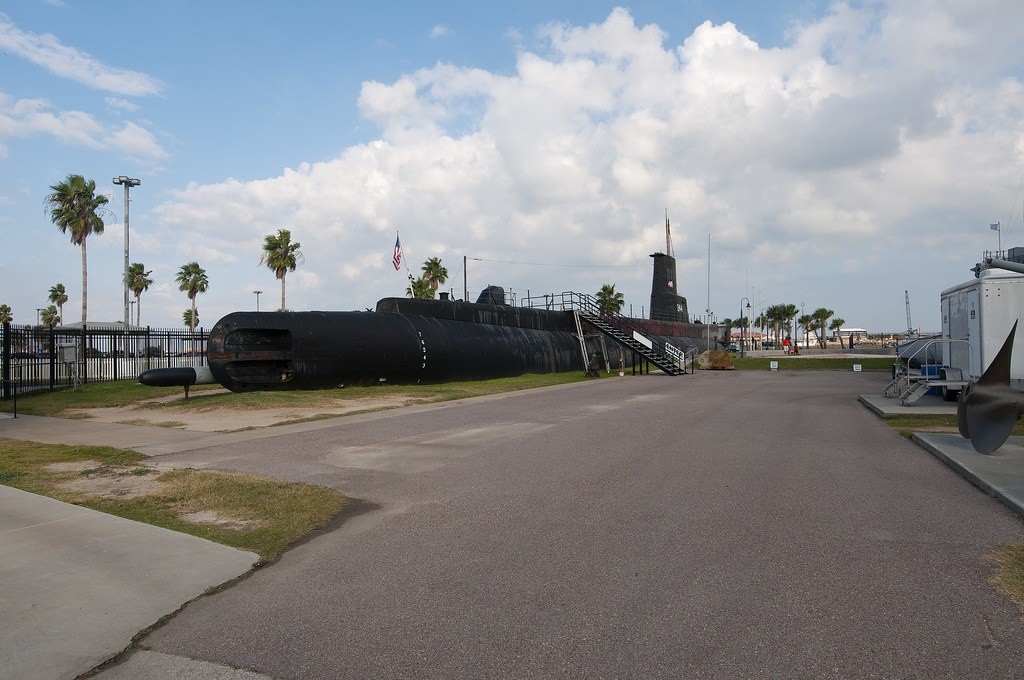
[990,224,1000,231]
[392,233,401,272]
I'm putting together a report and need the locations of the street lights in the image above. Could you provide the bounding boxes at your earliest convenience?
[740,298,751,358]
[113,176,141,330]
[253,291,263,312]
[129,301,136,325]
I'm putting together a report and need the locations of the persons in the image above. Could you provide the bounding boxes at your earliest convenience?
[783,337,799,355]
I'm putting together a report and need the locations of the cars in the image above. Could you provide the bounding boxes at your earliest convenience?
[883,342,894,348]
